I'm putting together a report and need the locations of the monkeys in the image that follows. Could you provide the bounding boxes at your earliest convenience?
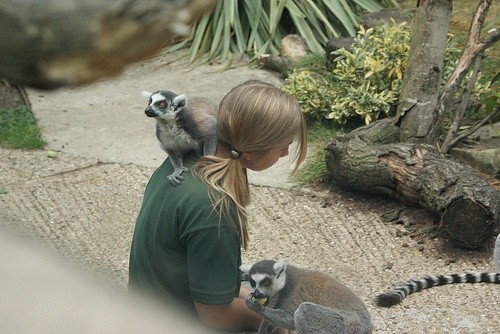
[143,88,218,186]
[238,259,373,334]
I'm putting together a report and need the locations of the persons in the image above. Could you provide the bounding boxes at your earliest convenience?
[127,79,309,334]
